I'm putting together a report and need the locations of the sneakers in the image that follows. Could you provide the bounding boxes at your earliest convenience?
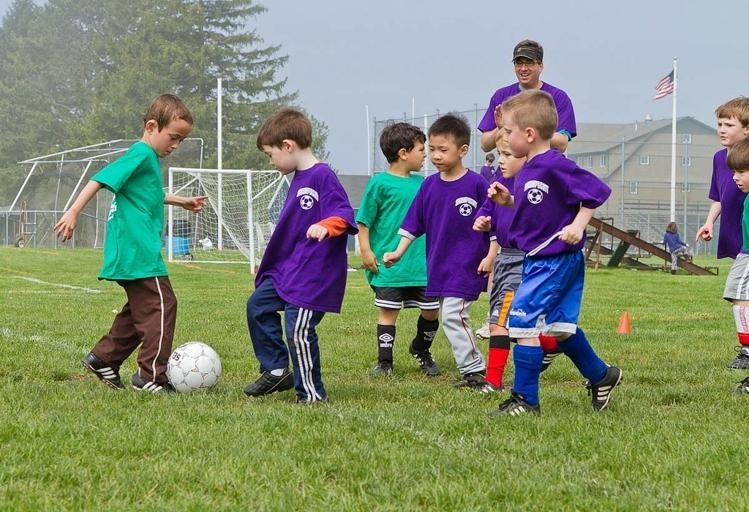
[736,376,749,395]
[590,365,622,411]
[242,370,294,397]
[367,361,396,378]
[453,370,504,395]
[726,345,747,370]
[81,351,125,391]
[406,339,441,376]
[130,370,173,395]
[488,393,543,419]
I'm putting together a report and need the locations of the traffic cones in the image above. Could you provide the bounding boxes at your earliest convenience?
[617,311,633,337]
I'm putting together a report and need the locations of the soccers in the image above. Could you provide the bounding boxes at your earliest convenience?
[166,342,221,394]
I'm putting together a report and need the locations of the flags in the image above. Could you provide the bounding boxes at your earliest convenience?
[654,68,675,101]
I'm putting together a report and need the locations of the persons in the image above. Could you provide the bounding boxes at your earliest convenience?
[655,221,689,275]
[480,153,495,182]
[471,126,528,399]
[726,137,749,395]
[474,40,577,340]
[383,114,493,392]
[500,89,623,418]
[53,93,209,396]
[695,95,749,260]
[355,121,443,377]
[243,109,358,407]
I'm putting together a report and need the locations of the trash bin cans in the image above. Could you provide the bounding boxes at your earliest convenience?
[166,220,191,254]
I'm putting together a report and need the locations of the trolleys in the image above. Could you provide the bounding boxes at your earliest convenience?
[14,200,29,249]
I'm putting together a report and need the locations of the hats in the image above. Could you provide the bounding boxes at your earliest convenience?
[511,39,545,63]
